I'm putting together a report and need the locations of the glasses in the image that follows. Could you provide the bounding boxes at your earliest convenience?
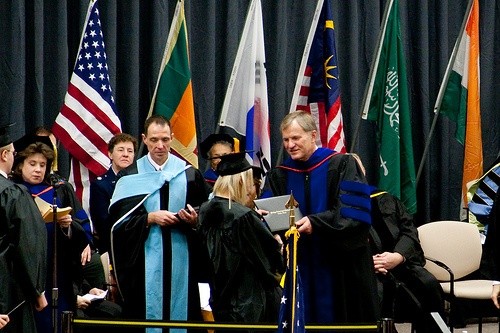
[8,150,17,156]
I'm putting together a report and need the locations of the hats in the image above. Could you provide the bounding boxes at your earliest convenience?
[0,121,16,148]
[199,133,235,159]
[15,133,53,151]
[207,150,255,176]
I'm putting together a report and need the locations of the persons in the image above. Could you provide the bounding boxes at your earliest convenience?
[480,184,500,333]
[255,109,382,332]
[90,115,212,332]
[0,121,122,333]
[199,132,285,333]
[346,152,447,333]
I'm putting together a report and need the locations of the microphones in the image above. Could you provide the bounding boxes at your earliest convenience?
[47,174,61,189]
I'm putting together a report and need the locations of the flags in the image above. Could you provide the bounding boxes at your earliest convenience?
[216,0,272,196]
[435,0,485,222]
[361,0,417,216]
[52,0,122,233]
[145,0,199,170]
[287,0,347,156]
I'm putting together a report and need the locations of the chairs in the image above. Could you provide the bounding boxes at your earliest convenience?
[417,220,500,333]
